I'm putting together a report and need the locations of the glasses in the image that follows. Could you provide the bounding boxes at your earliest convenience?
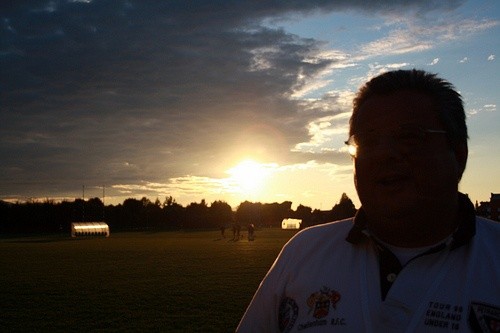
[343,133,454,159]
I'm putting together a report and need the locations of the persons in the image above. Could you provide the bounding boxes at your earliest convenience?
[219,223,255,240]
[234,68,500,333]
